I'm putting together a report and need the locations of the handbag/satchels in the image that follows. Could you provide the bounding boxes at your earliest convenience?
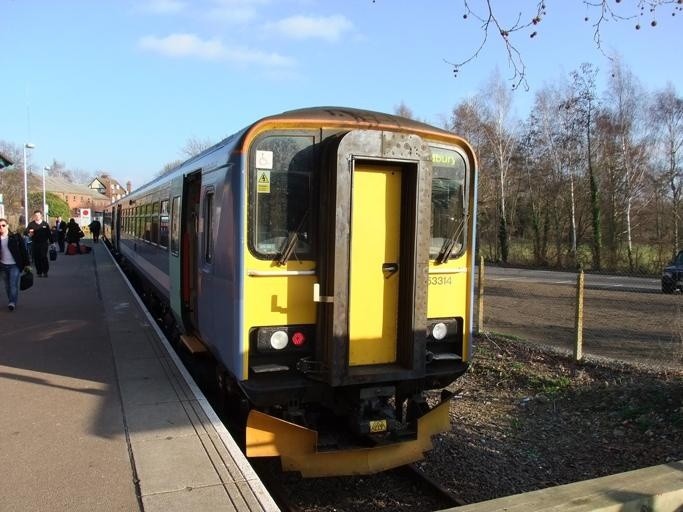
[49,247,57,261]
[20,270,33,291]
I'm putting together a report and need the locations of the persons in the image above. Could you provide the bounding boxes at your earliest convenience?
[54,216,67,252]
[23,210,53,277]
[92,217,100,243]
[65,218,82,255]
[0,219,31,312]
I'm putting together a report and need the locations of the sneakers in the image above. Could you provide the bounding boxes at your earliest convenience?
[8,302,15,312]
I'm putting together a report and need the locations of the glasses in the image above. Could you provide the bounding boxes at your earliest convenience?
[1,225,6,227]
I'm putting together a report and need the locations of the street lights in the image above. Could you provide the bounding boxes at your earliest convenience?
[23,143,50,228]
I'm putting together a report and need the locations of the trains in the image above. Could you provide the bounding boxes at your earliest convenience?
[102,106,478,479]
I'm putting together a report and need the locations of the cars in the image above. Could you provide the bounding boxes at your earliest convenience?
[662,250,683,293]
